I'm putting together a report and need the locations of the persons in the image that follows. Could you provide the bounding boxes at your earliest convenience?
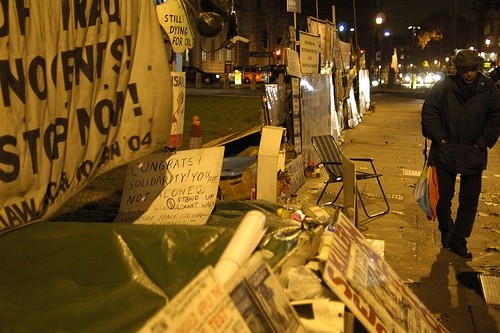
[421,50,500,259]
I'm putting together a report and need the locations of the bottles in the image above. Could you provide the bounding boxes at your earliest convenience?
[250,185,256,200]
[189,116,202,150]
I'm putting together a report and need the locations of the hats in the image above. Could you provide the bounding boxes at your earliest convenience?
[452,50,484,67]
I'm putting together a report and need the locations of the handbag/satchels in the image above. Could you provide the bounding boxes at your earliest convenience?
[414,138,440,222]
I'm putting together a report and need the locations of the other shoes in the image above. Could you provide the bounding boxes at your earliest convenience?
[451,244,472,258]
[441,227,453,248]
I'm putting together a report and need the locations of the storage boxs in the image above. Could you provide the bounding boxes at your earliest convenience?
[219,166,254,201]
[284,154,306,192]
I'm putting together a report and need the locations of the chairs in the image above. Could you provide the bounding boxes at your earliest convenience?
[311,135,390,219]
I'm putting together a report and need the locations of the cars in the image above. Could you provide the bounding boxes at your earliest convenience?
[231,64,291,84]
[182,67,221,84]
